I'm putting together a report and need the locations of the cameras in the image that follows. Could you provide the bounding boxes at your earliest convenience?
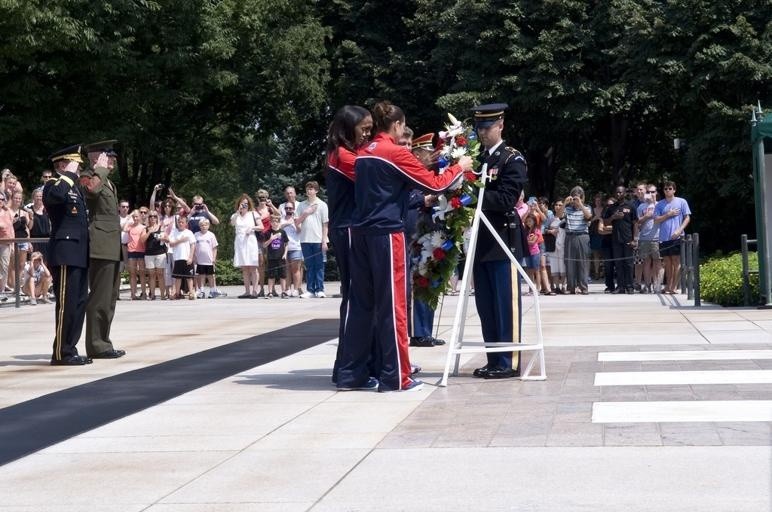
[242,204,248,208]
[196,206,203,210]
[644,194,652,198]
[570,197,576,202]
[286,211,294,216]
[529,197,535,203]
[159,184,166,189]
[260,198,266,202]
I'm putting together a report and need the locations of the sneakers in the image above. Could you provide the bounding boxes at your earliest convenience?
[127,288,303,300]
[300,291,315,298]
[527,282,679,296]
[336,377,379,391]
[0,288,55,307]
[317,291,326,298]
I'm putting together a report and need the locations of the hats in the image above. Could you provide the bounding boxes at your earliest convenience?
[48,143,84,163]
[85,140,119,157]
[411,132,436,153]
[470,103,509,129]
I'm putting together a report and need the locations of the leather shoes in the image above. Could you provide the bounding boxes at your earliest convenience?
[483,366,520,379]
[73,355,92,364]
[424,336,445,345]
[50,356,86,365]
[88,344,125,358]
[410,336,435,346]
[474,363,496,377]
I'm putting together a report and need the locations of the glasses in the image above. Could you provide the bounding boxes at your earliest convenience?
[122,206,129,208]
[646,191,656,194]
[140,211,146,214]
[149,215,157,217]
[0,198,5,201]
[164,204,170,207]
[664,188,672,190]
[42,175,52,178]
[284,207,292,210]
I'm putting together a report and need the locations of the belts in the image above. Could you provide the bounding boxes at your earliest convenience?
[566,232,587,235]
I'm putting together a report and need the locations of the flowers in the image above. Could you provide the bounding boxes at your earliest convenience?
[404,112,484,310]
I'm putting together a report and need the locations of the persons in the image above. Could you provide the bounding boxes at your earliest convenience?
[472,97,530,381]
[324,106,369,381]
[514,178,693,297]
[350,103,377,149]
[399,128,466,347]
[42,142,96,366]
[333,100,474,392]
[118,184,221,300]
[86,137,125,359]
[0,167,53,305]
[230,180,331,299]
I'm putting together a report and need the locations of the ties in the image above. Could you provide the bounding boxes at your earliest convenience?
[481,149,489,164]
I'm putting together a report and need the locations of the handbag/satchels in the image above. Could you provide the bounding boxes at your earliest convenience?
[542,233,556,251]
